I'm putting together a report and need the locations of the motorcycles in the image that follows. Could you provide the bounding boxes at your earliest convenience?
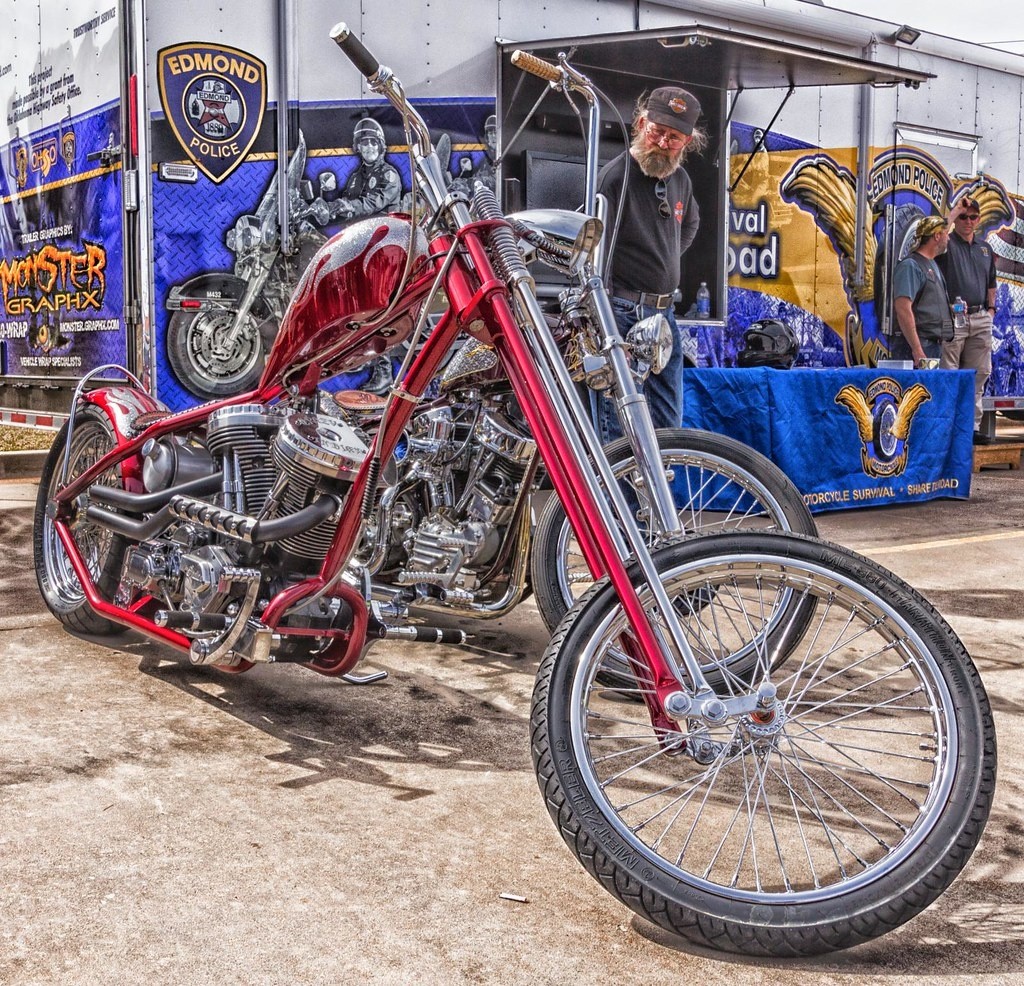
[36,20,998,959]
[168,126,473,402]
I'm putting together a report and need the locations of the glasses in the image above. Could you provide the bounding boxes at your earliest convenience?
[655,179,671,219]
[928,218,948,230]
[959,214,978,220]
[645,122,689,150]
[359,138,378,146]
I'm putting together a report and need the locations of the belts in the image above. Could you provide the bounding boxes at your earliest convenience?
[613,286,673,309]
[927,337,943,344]
[968,305,984,314]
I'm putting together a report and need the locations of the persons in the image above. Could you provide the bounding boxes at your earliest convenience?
[934,197,996,444]
[593,85,702,550]
[894,215,967,368]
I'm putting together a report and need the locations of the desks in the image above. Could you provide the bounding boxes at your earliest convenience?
[666,365,978,517]
[971,439,1024,477]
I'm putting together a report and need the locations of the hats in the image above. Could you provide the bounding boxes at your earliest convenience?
[647,86,702,135]
[963,198,979,213]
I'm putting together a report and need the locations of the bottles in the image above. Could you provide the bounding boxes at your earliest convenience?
[953,296,966,329]
[696,282,710,319]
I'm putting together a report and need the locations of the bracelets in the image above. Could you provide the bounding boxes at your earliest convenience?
[988,307,996,313]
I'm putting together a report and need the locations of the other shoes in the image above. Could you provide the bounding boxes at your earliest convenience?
[647,584,720,617]
[973,431,991,445]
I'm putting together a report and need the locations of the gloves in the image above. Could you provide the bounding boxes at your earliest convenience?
[327,198,355,219]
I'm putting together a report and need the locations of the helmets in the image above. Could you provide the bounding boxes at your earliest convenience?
[352,117,387,153]
[737,319,799,369]
